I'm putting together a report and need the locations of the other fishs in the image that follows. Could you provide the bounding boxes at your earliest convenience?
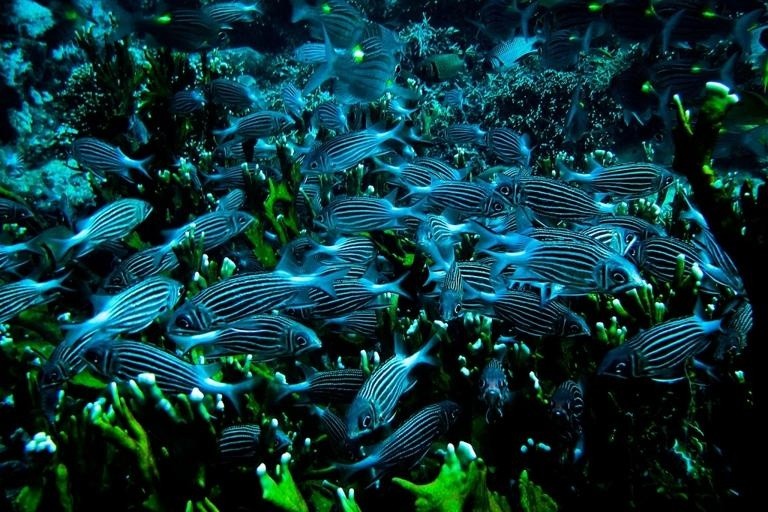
[0,0,768,512]
